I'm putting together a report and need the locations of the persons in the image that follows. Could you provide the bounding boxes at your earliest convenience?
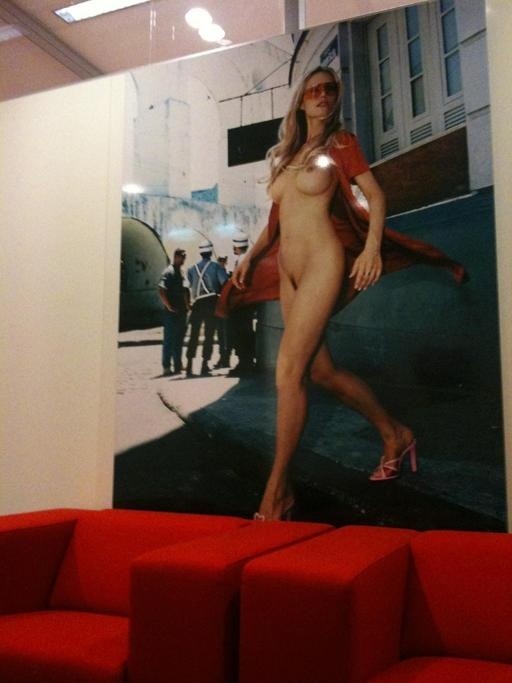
[211,253,234,368]
[226,232,255,378]
[227,61,468,523]
[187,238,227,375]
[155,246,191,372]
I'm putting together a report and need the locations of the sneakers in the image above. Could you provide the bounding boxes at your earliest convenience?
[163,358,251,378]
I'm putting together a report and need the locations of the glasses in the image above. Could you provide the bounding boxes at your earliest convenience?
[302,80,338,99]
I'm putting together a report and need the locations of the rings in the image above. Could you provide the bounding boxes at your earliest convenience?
[364,271,370,275]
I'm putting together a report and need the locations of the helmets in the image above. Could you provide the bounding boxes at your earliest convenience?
[232,231,251,248]
[198,241,213,253]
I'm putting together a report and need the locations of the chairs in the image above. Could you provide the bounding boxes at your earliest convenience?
[0,506,512,683]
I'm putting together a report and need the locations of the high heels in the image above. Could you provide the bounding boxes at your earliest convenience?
[253,490,298,521]
[368,438,418,481]
